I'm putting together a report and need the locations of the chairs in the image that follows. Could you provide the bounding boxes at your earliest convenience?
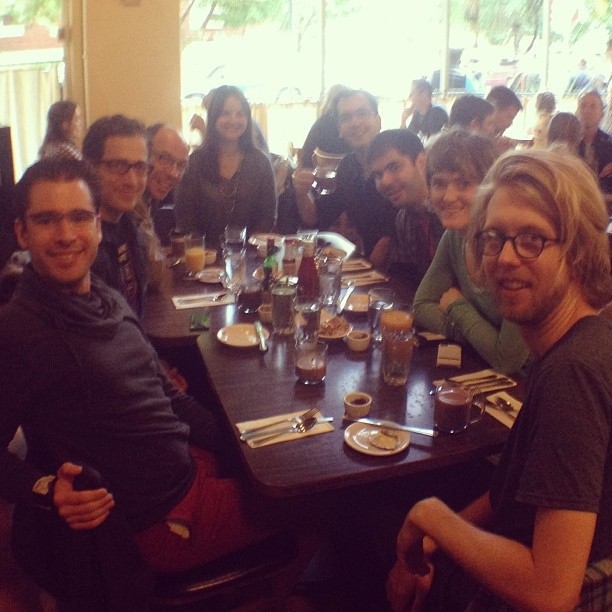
[11,438,299,610]
[432,554,612,612]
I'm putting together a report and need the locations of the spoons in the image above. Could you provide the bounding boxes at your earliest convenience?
[347,279,387,286]
[493,396,519,414]
[178,294,227,306]
[253,417,317,445]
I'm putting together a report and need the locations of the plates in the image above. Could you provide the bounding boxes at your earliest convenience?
[318,317,354,340]
[249,233,281,245]
[217,323,269,348]
[343,418,411,456]
[195,267,225,284]
[337,292,381,312]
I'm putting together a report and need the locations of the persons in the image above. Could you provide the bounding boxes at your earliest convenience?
[190,91,270,150]
[485,85,524,150]
[79,115,189,396]
[448,95,497,139]
[399,79,448,142]
[135,120,190,219]
[575,92,612,195]
[531,92,556,147]
[543,111,580,149]
[292,89,399,262]
[384,149,612,609]
[37,100,85,163]
[173,85,279,246]
[500,57,529,96]
[408,130,531,376]
[294,83,354,162]
[367,129,445,285]
[0,158,305,577]
[430,47,475,98]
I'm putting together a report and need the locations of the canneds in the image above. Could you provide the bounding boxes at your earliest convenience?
[271,287,298,336]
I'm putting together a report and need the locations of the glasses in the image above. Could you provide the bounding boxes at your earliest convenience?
[335,106,375,124]
[89,159,152,178]
[149,150,189,175]
[473,226,568,260]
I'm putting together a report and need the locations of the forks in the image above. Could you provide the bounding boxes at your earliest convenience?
[474,392,516,421]
[241,405,320,434]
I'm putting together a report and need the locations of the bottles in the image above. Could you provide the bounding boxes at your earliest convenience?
[262,238,279,287]
[297,241,320,302]
[282,239,295,274]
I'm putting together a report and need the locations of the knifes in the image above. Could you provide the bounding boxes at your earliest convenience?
[341,415,439,438]
[254,319,268,352]
[240,416,334,441]
[336,283,356,315]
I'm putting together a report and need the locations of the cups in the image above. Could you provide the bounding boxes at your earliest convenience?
[367,286,397,340]
[235,284,264,313]
[202,248,218,265]
[381,326,415,385]
[379,310,416,332]
[347,331,370,352]
[255,245,268,257]
[292,296,321,349]
[256,304,275,323]
[219,225,248,288]
[321,258,342,311]
[269,282,297,336]
[311,168,339,195]
[344,392,372,416]
[434,384,485,435]
[293,341,328,384]
[183,232,207,271]
